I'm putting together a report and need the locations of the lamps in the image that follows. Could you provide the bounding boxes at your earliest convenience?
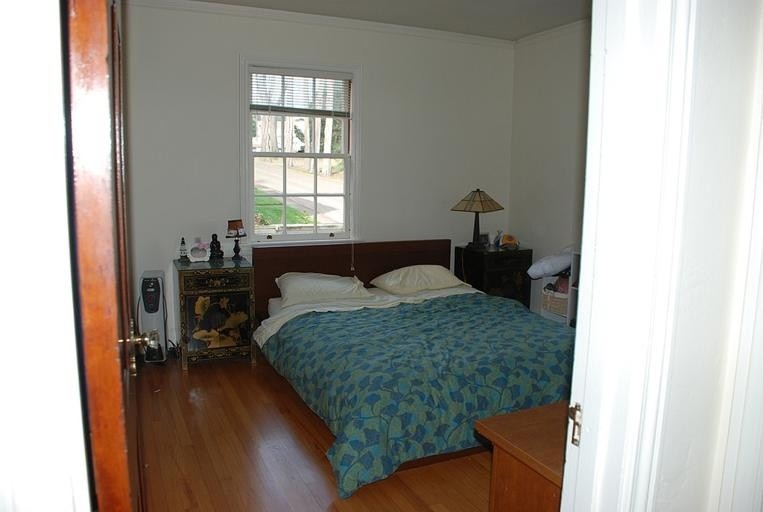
[227,220,249,260]
[450,188,505,247]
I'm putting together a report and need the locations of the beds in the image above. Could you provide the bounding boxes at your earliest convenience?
[251,238,576,462]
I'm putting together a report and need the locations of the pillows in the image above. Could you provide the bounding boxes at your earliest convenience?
[274,262,467,310]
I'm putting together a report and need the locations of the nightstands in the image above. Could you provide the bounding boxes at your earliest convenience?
[173,259,256,372]
[454,244,533,313]
